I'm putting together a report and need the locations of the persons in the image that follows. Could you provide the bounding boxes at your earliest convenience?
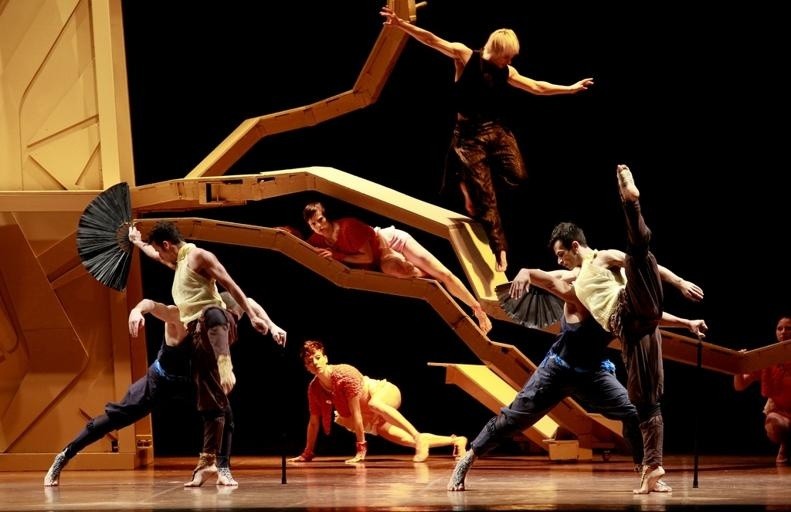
[548,165,705,495]
[380,4,598,272]
[44,289,288,488]
[127,220,270,489]
[269,199,494,340]
[446,269,708,489]
[286,340,468,463]
[733,315,791,466]
